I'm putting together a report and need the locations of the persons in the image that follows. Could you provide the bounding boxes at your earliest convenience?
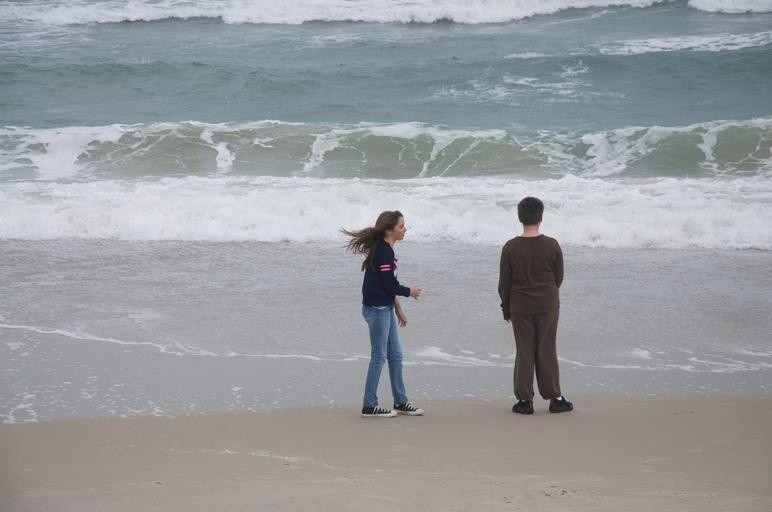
[338,210,424,416]
[497,196,575,412]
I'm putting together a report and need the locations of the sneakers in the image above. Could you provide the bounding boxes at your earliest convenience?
[361,406,396,417]
[512,399,534,414]
[393,402,424,416]
[549,395,573,412]
